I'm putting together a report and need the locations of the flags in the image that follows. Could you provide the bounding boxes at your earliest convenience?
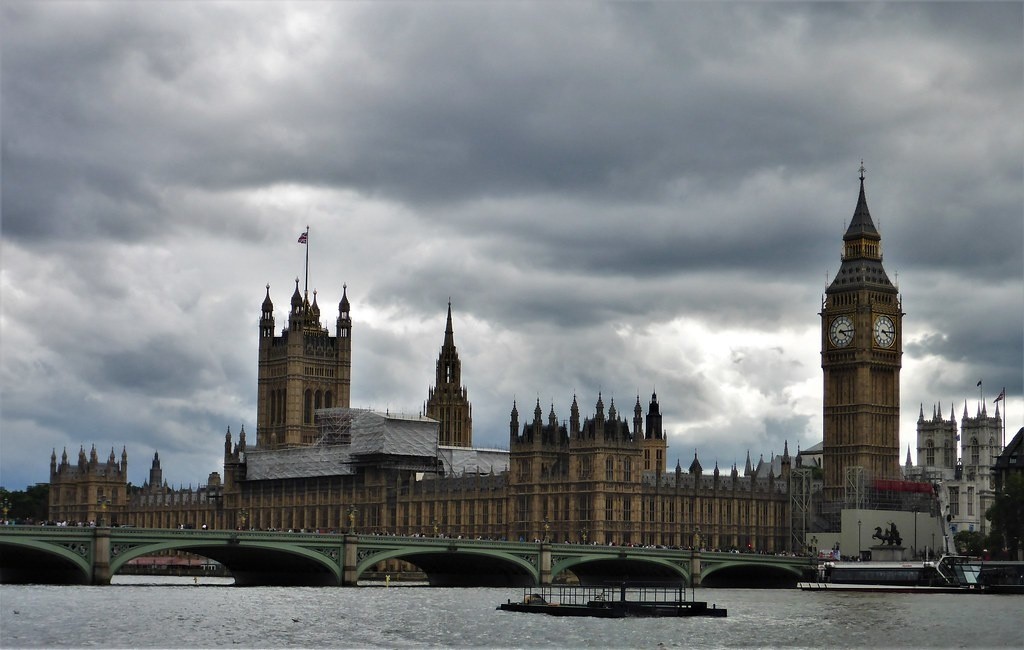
[993,388,1005,403]
[977,381,982,387]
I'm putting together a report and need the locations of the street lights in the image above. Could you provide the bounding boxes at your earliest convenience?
[857,519,862,560]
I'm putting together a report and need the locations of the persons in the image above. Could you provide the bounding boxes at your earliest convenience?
[372,529,543,543]
[178,523,209,530]
[237,526,343,534]
[564,534,865,562]
[0,517,127,528]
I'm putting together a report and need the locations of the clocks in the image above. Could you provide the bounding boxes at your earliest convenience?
[873,315,896,348]
[828,314,855,348]
